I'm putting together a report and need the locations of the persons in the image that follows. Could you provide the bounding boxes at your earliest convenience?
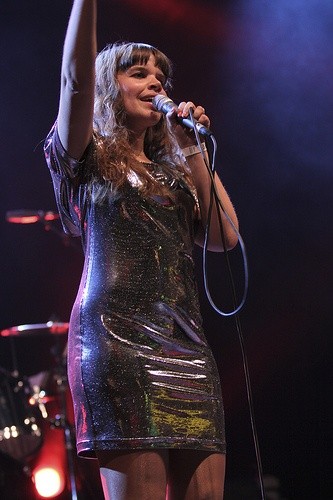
[43,0,239,499]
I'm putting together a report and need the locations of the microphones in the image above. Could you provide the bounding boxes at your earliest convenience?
[153,94,211,138]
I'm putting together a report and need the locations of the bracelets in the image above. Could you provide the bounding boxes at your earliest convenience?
[181,142,206,158]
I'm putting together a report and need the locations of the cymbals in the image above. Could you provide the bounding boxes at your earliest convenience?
[1,209,60,225]
[0,322,70,339]
